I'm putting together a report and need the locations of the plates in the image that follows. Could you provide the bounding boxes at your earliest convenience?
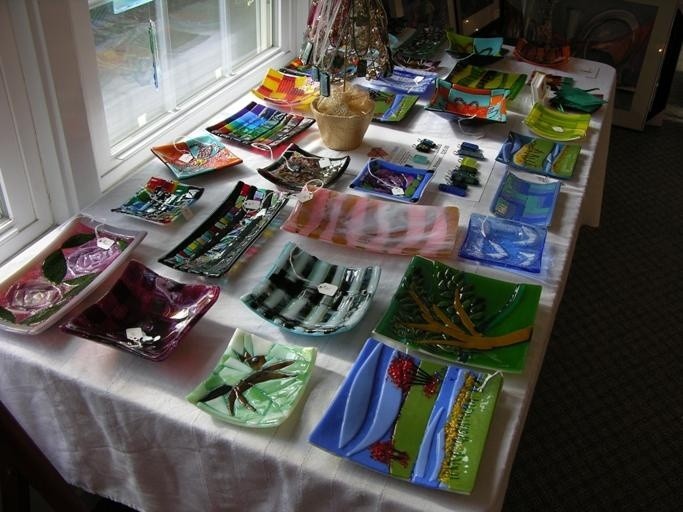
[185,326,319,430]
[203,99,317,155]
[369,254,544,375]
[109,175,205,230]
[372,69,435,97]
[149,131,244,182]
[456,212,548,276]
[1,212,150,336]
[239,241,381,338]
[302,21,401,86]
[309,337,506,497]
[57,257,222,363]
[489,169,563,228]
[251,68,321,108]
[493,130,583,181]
[359,84,419,124]
[393,22,607,140]
[156,182,301,280]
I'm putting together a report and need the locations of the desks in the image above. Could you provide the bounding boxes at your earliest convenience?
[0,52,619,512]
